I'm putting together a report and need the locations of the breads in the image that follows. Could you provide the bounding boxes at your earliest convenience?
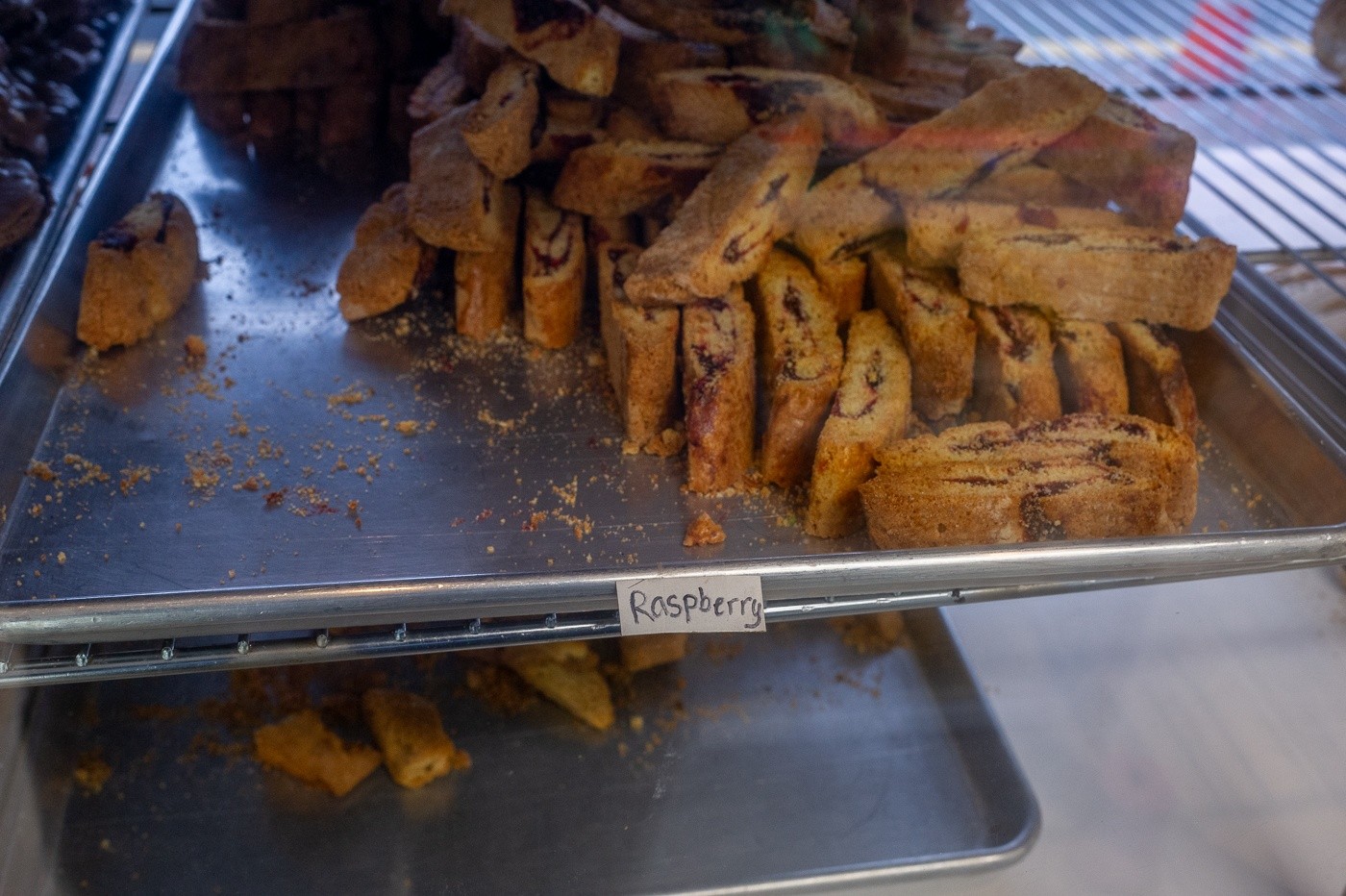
[258,606,908,790]
[72,0,1240,546]
